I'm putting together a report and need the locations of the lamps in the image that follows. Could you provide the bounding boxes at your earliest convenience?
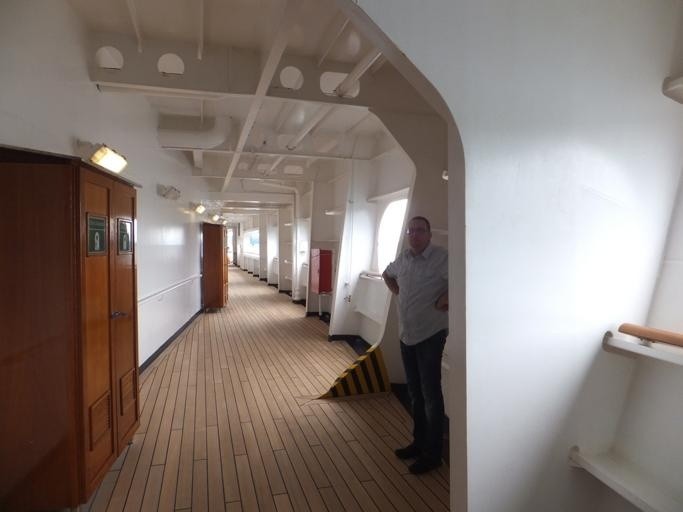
[161,185,181,202]
[79,142,129,174]
[190,200,205,214]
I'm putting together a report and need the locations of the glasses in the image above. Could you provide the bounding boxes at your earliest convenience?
[405,225,430,235]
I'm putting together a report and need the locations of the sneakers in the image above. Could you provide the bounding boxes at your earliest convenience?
[406,453,445,476]
[394,438,425,460]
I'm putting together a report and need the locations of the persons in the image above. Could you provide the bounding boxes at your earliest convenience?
[381,216,448,477]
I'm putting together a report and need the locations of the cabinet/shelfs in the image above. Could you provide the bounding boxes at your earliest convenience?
[269,174,451,383]
[573,72,682,510]
[202,221,229,314]
[0,144,142,505]
